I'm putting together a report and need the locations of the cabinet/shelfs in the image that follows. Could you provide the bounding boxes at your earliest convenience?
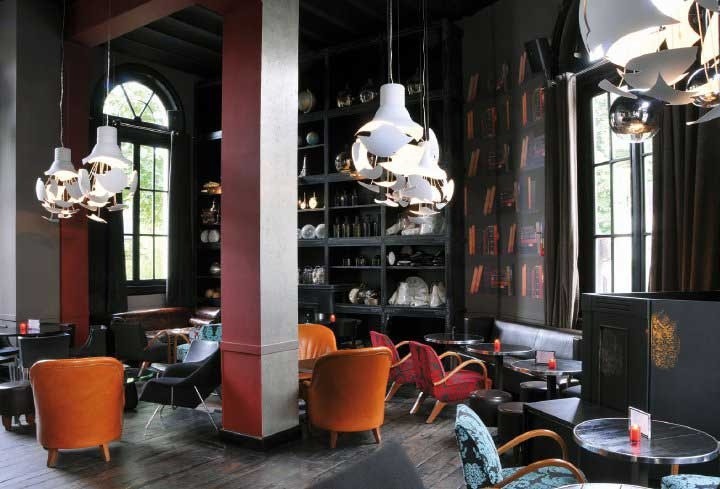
[197,27,463,385]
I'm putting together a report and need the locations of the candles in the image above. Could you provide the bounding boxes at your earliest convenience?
[630,426,640,441]
[331,314,335,322]
[549,359,556,368]
[495,339,500,349]
[20,323,25,329]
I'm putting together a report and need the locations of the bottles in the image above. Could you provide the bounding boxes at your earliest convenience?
[331,190,358,206]
[299,266,325,284]
[396,281,410,304]
[343,253,380,267]
[332,214,381,238]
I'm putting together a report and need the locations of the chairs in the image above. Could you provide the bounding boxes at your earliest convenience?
[0,307,720,489]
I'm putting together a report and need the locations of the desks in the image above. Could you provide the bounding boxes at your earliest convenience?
[466,343,532,391]
[0,326,72,379]
[573,418,720,489]
[425,332,483,370]
[511,359,583,400]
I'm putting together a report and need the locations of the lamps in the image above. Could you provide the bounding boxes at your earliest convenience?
[35,0,138,224]
[352,0,454,224]
[579,0,720,126]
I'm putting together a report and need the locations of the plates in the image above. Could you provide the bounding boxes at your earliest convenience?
[201,229,219,243]
[296,223,325,240]
[405,276,428,306]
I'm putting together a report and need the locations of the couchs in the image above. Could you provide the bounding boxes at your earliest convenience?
[456,315,583,402]
